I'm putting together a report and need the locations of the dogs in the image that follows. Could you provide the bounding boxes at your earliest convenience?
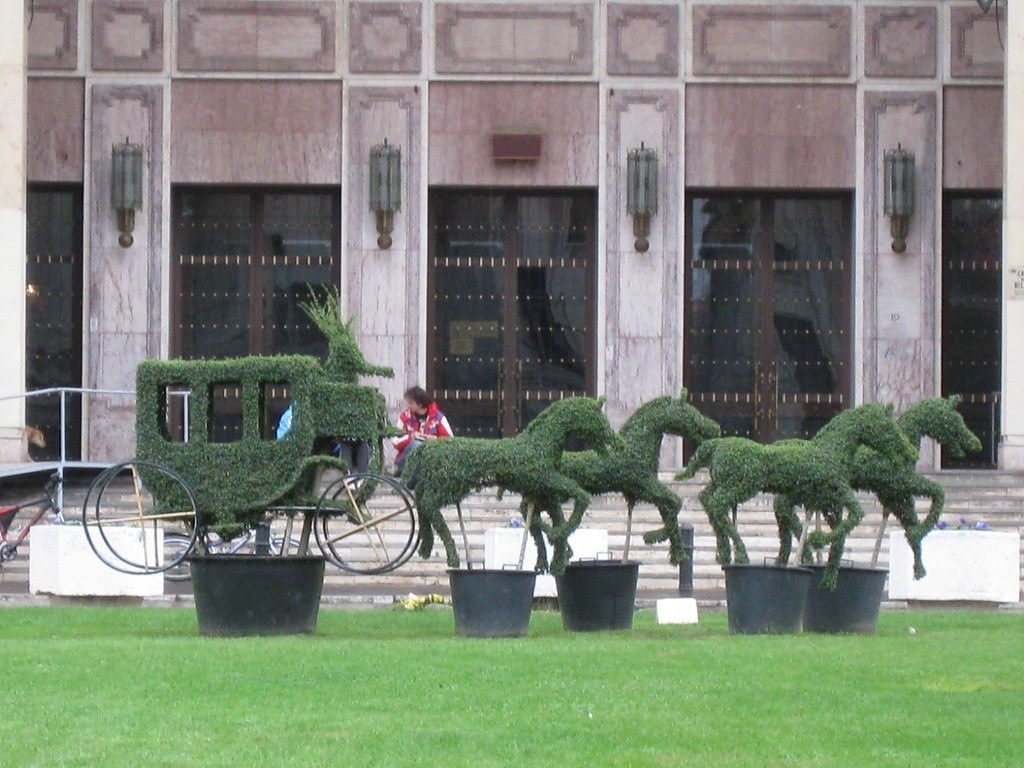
[25,425,46,461]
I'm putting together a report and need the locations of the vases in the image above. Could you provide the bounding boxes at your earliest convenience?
[180,555,331,633]
[720,565,814,635]
[801,563,890,635]
[27,522,166,598]
[444,569,543,639]
[552,560,644,630]
[887,527,1020,604]
[483,526,610,598]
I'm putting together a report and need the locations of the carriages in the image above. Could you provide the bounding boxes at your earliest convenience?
[81,280,985,576]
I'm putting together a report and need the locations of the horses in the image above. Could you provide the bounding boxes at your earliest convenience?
[396,395,983,593]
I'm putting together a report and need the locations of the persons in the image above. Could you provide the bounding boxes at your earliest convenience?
[331,436,370,494]
[274,402,292,441]
[386,386,454,477]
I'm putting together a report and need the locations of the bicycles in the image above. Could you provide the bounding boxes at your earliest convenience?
[0,472,68,565]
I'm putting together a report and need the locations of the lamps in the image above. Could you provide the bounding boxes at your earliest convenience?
[368,138,403,250]
[882,142,918,255]
[626,141,660,254]
[112,136,144,249]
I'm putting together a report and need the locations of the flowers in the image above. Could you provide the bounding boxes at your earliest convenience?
[53,516,141,527]
[935,515,994,531]
[500,518,523,528]
